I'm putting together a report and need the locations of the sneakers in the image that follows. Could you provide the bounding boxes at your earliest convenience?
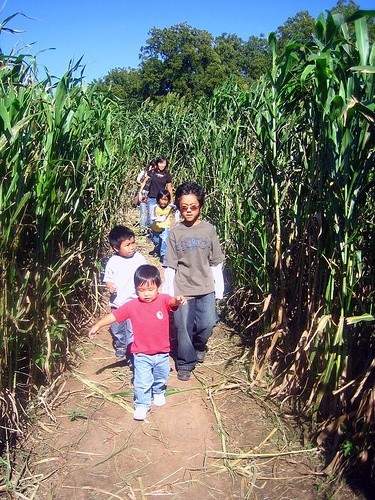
[134,408,148,420]
[154,393,166,406]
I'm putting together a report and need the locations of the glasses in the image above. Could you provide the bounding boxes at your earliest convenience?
[180,205,200,211]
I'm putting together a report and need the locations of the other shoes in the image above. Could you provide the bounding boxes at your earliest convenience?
[197,351,205,361]
[129,359,134,367]
[116,349,127,357]
[177,370,190,380]
[153,251,160,257]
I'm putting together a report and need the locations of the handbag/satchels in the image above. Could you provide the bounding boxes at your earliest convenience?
[144,170,155,191]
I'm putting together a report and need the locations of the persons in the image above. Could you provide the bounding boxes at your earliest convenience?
[150,190,181,267]
[87,265,187,421]
[162,181,225,381]
[136,156,174,237]
[103,225,147,370]
